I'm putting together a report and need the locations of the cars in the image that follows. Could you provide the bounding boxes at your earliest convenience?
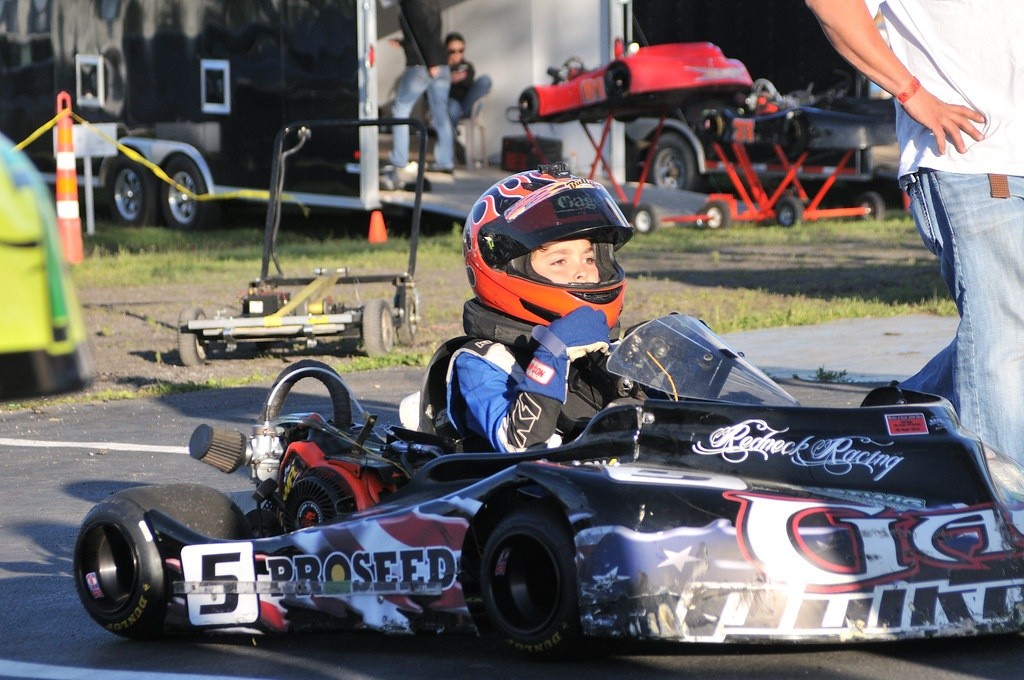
[640,98,910,222]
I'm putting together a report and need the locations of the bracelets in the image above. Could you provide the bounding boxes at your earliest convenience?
[896,76,921,102]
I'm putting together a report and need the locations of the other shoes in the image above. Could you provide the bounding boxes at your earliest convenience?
[428,165,452,174]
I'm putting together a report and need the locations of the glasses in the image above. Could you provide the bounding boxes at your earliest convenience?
[450,48,465,55]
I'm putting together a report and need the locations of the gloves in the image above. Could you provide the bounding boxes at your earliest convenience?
[513,305,615,405]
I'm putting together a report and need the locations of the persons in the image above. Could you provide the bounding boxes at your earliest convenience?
[381,0,455,175]
[443,165,693,453]
[424,31,492,129]
[805,0,1024,472]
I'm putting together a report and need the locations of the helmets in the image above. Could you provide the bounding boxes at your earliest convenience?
[461,161,635,330]
